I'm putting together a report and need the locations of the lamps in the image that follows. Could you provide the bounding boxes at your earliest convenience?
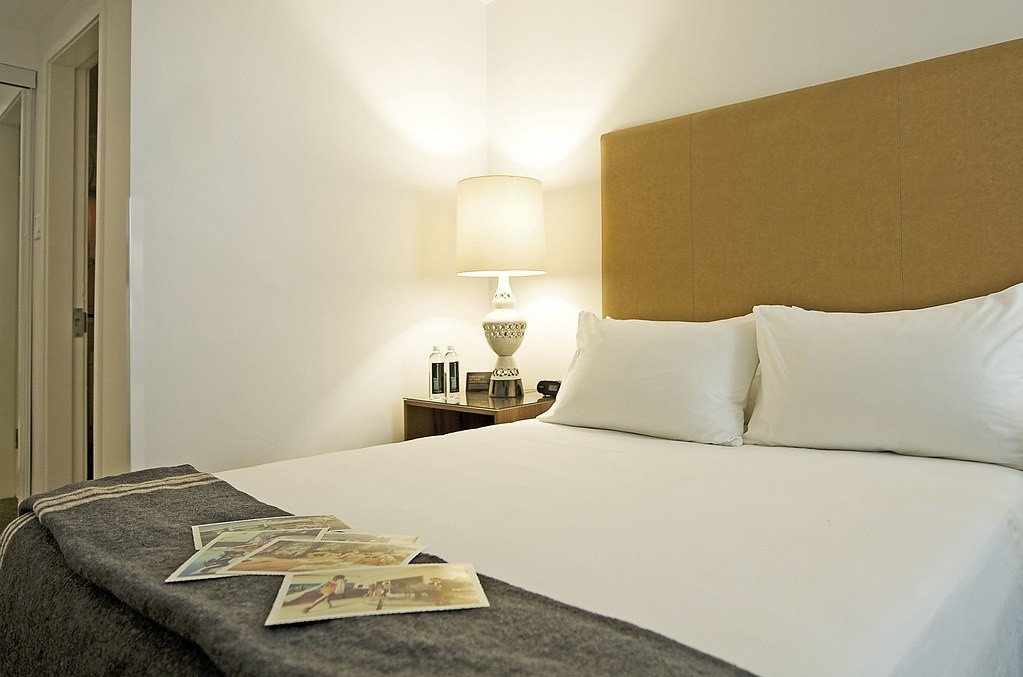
[455,174,551,396]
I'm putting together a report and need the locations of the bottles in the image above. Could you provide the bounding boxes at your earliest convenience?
[428,345,444,400]
[444,346,460,399]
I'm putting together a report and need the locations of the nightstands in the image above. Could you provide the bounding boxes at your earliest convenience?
[402,386,556,443]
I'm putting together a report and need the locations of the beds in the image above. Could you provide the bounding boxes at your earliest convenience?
[0,37,1021,676]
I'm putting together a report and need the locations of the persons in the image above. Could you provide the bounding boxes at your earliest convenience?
[302,574,345,613]
[381,582,391,598]
[363,584,375,599]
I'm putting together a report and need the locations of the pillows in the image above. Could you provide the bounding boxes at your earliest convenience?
[742,280,1023,471]
[536,311,759,447]
